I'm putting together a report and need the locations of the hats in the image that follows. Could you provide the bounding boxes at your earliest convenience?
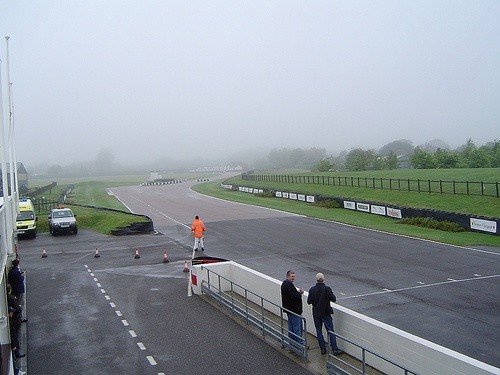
[316,273,324,281]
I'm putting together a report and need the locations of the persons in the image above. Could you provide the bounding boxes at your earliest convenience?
[307,273,342,355]
[281,270,304,351]
[191,216,206,251]
[6,259,26,375]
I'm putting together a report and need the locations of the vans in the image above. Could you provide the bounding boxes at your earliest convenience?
[16,198,38,239]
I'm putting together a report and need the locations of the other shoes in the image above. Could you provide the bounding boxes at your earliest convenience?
[195,248,198,251]
[321,350,327,354]
[18,370,26,375]
[202,247,204,251]
[334,350,342,355]
[18,354,25,358]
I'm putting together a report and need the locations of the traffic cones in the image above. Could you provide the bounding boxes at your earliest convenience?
[183,260,190,272]
[41,248,48,258]
[94,248,100,258]
[134,248,140,259]
[162,251,169,263]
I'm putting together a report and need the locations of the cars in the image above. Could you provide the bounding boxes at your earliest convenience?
[48,207,78,236]
